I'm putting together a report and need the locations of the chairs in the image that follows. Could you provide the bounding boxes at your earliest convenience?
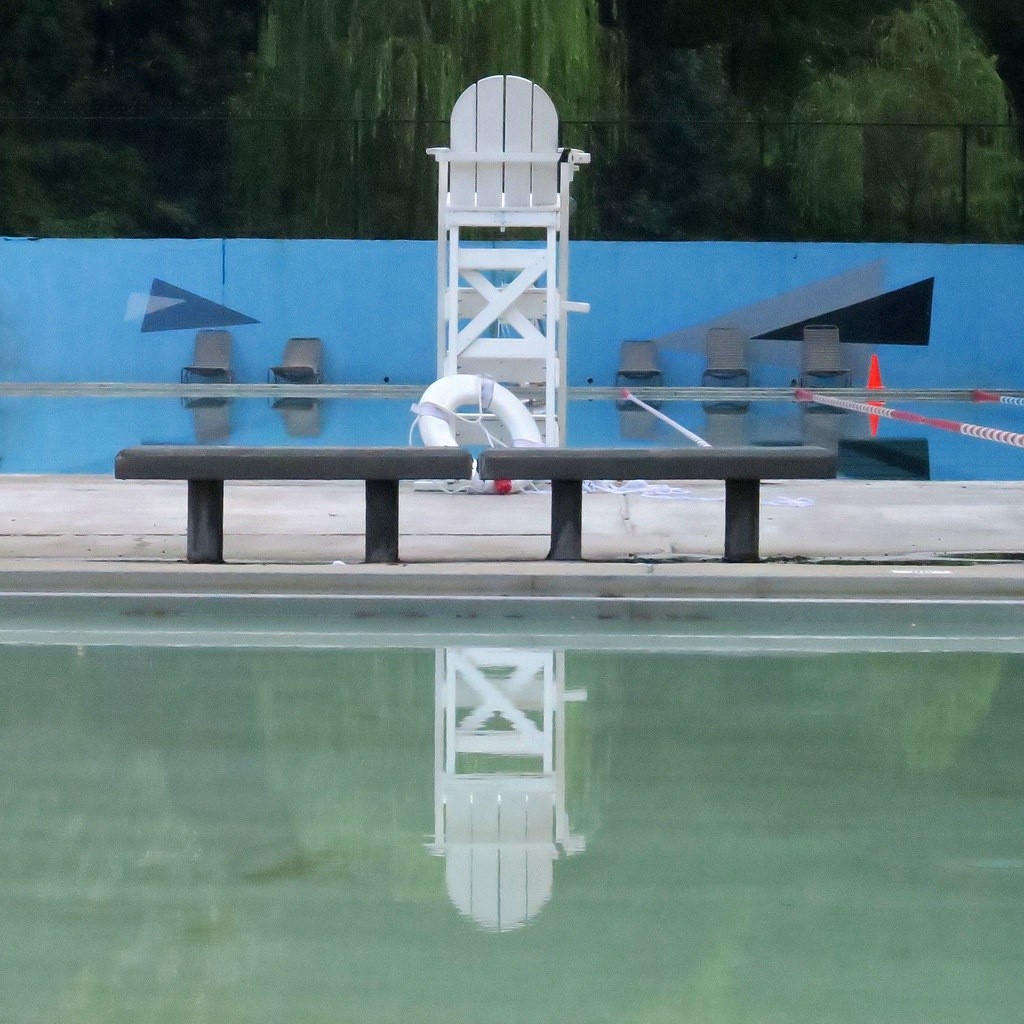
[614,337,663,389]
[180,325,233,382]
[270,395,324,438]
[427,74,593,399]
[178,397,233,442]
[615,401,665,441]
[422,646,589,936]
[701,326,753,389]
[267,333,323,384]
[798,401,853,459]
[798,322,852,388]
[701,400,756,448]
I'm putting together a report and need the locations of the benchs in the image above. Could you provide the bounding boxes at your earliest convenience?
[477,437,840,569]
[114,445,472,564]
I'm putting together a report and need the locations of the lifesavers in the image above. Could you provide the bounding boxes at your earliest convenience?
[416,373,542,496]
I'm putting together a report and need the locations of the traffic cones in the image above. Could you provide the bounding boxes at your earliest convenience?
[866,354,885,389]
[868,400,886,437]
